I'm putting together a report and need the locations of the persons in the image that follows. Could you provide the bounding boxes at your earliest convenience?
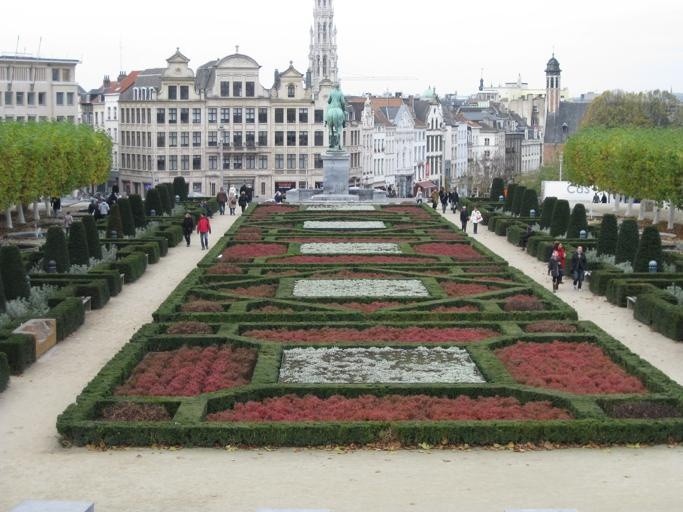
[460,206,469,233]
[217,182,254,216]
[182,212,195,247]
[51,198,60,218]
[274,189,282,203]
[197,213,211,250]
[592,193,600,203]
[415,186,422,204]
[547,242,586,292]
[63,211,73,236]
[601,193,607,203]
[431,188,459,214]
[324,82,346,129]
[471,207,481,234]
[88,190,121,220]
